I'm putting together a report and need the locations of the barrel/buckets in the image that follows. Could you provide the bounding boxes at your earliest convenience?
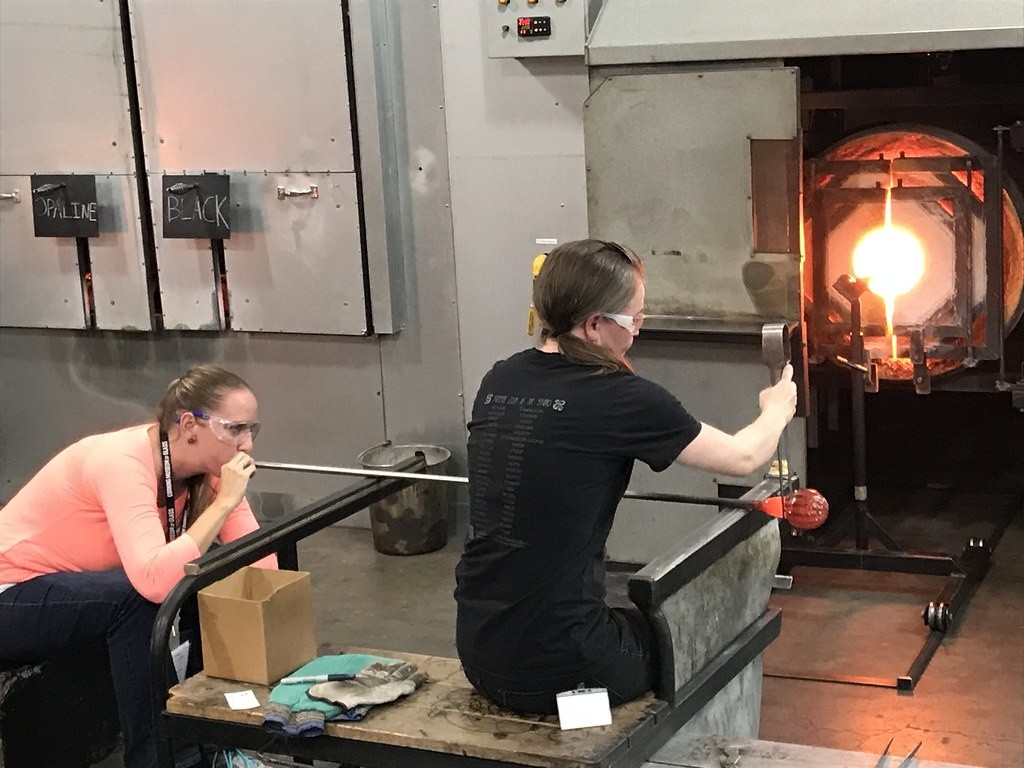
[358,440,452,556]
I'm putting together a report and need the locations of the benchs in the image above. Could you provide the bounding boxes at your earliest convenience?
[160,645,669,768]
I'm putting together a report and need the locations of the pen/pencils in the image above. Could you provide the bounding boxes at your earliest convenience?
[280,672,356,683]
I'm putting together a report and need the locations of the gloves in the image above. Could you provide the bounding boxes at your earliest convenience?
[307,661,430,711]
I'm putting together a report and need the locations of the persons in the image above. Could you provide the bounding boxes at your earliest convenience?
[454,239,798,714]
[0,364,281,768]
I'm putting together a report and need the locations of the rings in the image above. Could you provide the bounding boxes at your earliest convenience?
[238,461,245,466]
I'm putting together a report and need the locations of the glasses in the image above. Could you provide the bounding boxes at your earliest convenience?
[176,410,261,445]
[603,313,644,336]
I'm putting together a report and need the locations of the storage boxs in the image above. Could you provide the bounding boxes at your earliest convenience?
[197,566,317,686]
